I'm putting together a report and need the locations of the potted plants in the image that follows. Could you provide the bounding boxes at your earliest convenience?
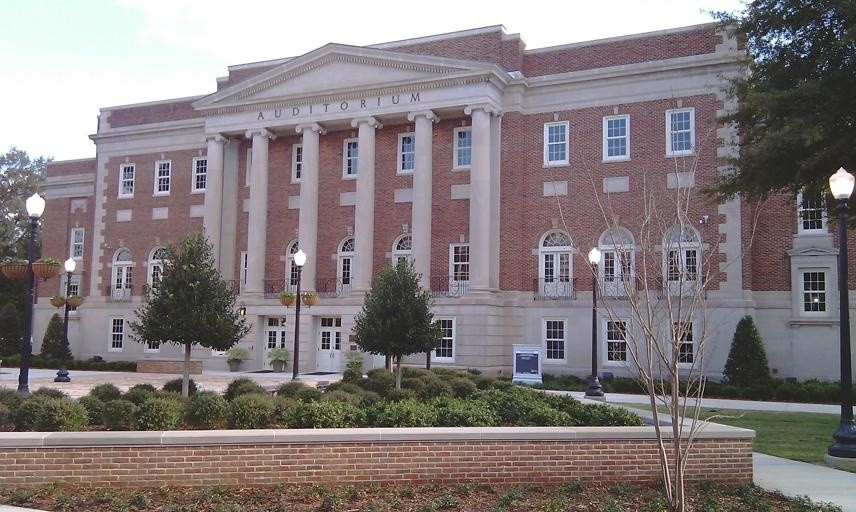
[225,347,247,372]
[1,258,62,281]
[266,348,289,372]
[50,295,83,308]
[278,291,318,308]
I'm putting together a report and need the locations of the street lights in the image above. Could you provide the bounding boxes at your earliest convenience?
[54,257,76,382]
[828,166,856,458]
[15,192,46,399]
[585,247,604,397]
[291,249,306,381]
